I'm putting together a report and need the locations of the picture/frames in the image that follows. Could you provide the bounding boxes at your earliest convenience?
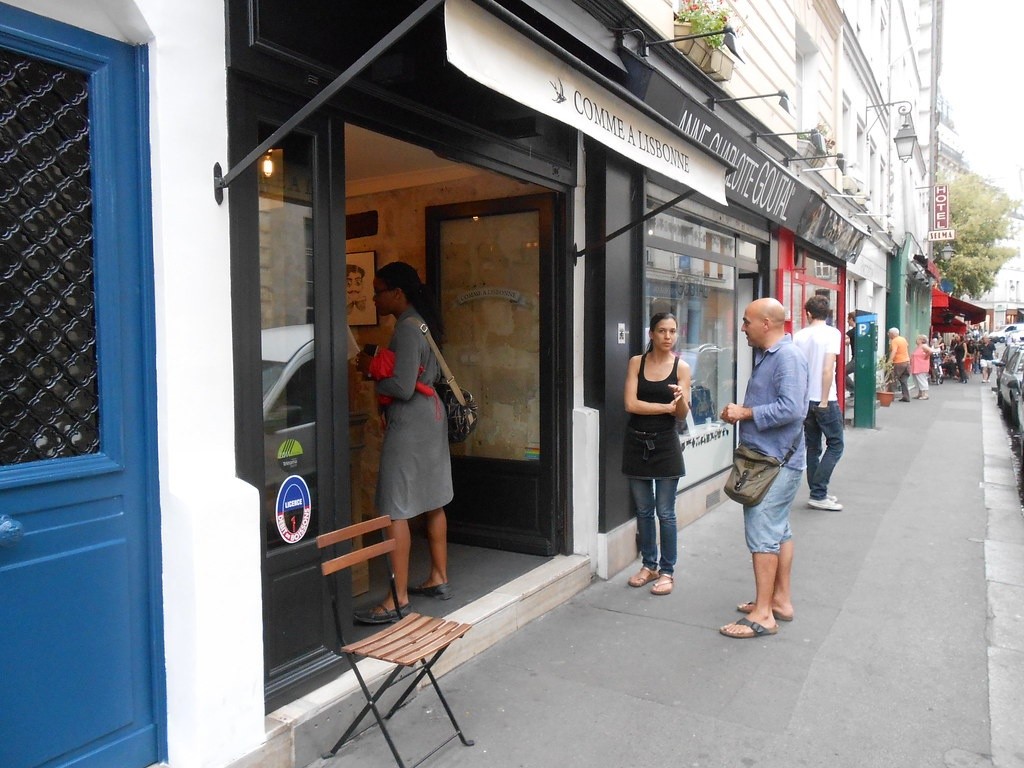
[345,250,379,327]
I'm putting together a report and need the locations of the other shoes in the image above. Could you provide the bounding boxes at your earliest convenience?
[808,498,843,510]
[826,494,837,503]
[912,396,920,399]
[919,396,928,400]
[352,603,412,622]
[408,583,453,600]
[899,399,910,402]
[846,393,855,400]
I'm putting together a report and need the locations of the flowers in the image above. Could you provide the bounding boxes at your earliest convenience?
[796,123,836,154]
[675,0,748,50]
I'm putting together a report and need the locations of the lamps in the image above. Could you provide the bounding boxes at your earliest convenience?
[706,90,791,114]
[620,27,744,63]
[865,100,918,163]
[782,153,846,175]
[263,149,273,177]
[750,129,828,156]
[912,271,930,288]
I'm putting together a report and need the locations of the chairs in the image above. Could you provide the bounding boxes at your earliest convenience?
[316,515,474,768]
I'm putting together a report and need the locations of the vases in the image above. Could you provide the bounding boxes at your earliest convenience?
[672,21,713,68]
[701,46,736,83]
[797,139,827,170]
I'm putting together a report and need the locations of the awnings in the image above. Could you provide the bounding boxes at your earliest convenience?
[213,0,742,258]
[932,286,986,325]
[930,317,966,333]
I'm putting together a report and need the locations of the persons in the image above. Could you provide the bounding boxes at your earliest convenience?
[886,327,912,402]
[846,312,862,400]
[911,334,932,400]
[795,293,845,512]
[352,262,454,625]
[924,328,999,387]
[719,297,810,638]
[623,312,691,596]
[346,321,360,370]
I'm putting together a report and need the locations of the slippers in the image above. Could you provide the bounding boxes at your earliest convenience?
[737,601,793,620]
[720,617,779,638]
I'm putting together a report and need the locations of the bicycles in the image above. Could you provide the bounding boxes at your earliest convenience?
[932,352,945,385]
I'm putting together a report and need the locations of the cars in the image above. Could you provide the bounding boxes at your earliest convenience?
[257,324,317,537]
[993,323,1024,435]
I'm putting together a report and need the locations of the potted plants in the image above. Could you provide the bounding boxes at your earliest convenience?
[876,354,897,407]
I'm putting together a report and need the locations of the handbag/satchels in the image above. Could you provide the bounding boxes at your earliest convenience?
[439,383,478,442]
[724,445,780,506]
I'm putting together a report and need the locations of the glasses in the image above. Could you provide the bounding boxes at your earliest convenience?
[373,287,398,296]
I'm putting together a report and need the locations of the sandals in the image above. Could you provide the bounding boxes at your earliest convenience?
[652,574,674,595]
[629,567,657,586]
